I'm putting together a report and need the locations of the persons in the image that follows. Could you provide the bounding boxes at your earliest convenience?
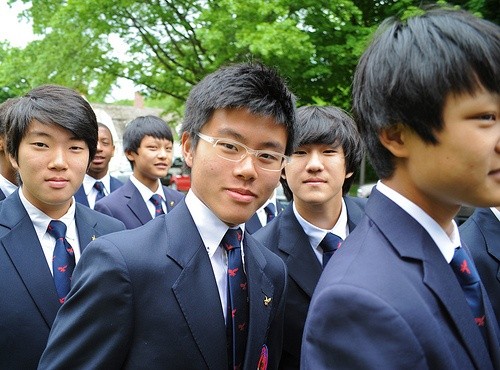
[250,104,370,370]
[299,7,500,370]
[94,115,186,228]
[74,122,124,209]
[244,188,285,235]
[458,206,500,322]
[37,62,296,370]
[0,86,126,369]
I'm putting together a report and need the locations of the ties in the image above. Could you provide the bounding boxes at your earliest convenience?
[220,225,251,370]
[47,220,76,308]
[93,181,109,203]
[449,247,490,356]
[263,203,278,224]
[319,232,343,270]
[149,194,165,218]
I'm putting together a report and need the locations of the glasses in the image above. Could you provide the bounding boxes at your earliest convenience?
[198,132,292,173]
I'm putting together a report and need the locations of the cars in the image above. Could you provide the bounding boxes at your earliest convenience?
[170,157,184,168]
[169,167,191,191]
[161,173,174,186]
